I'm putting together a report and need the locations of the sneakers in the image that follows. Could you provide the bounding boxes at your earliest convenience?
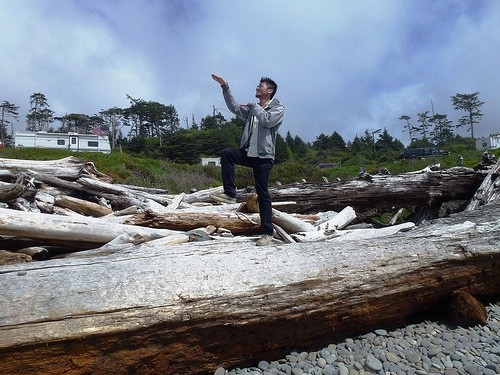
[256,234,274,246]
[210,192,238,204]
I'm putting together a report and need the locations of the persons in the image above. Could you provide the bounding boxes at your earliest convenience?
[211,73,286,246]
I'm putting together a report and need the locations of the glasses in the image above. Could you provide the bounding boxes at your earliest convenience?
[257,84,269,89]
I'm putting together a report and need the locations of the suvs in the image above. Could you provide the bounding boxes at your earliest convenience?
[399,147,451,162]
